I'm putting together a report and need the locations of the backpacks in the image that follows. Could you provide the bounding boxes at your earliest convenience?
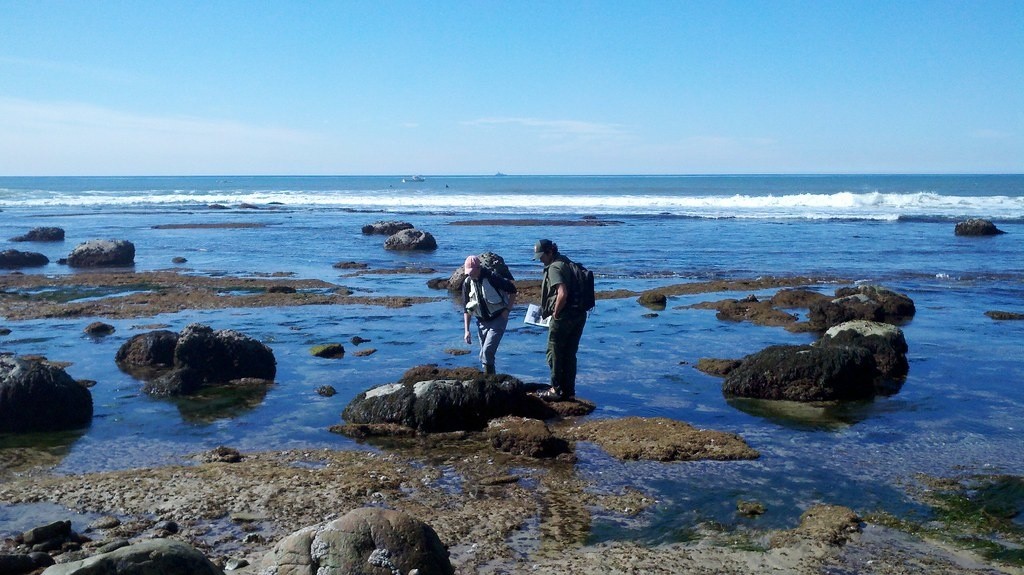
[543,258,596,317]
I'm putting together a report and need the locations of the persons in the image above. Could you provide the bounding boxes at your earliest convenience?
[461,250,517,376]
[528,238,588,402]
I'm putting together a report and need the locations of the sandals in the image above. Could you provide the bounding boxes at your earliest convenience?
[538,385,576,402]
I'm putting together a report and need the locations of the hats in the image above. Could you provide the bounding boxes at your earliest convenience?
[534,239,552,259]
[464,255,480,276]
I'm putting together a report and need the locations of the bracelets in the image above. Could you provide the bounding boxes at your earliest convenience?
[505,306,511,313]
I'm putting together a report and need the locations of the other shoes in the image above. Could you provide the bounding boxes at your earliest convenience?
[482,363,496,375]
